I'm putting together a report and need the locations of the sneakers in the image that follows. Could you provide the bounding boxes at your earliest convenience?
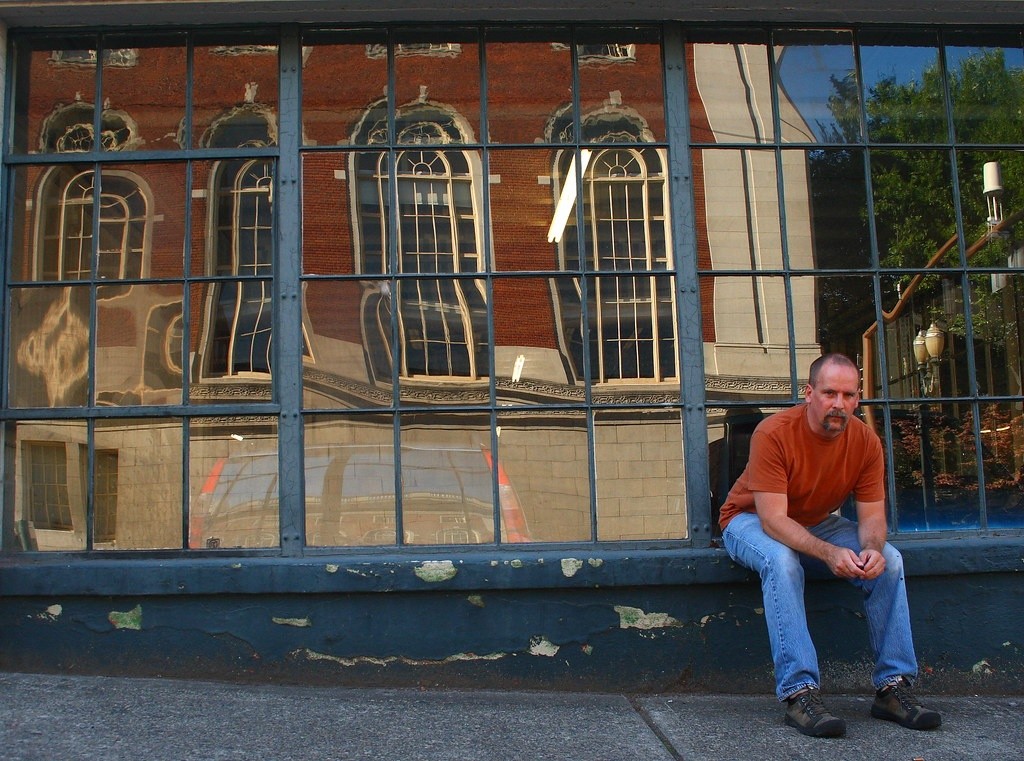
[870,680,942,732]
[784,692,846,738]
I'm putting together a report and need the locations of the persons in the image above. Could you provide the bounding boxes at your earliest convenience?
[719,353,941,736]
